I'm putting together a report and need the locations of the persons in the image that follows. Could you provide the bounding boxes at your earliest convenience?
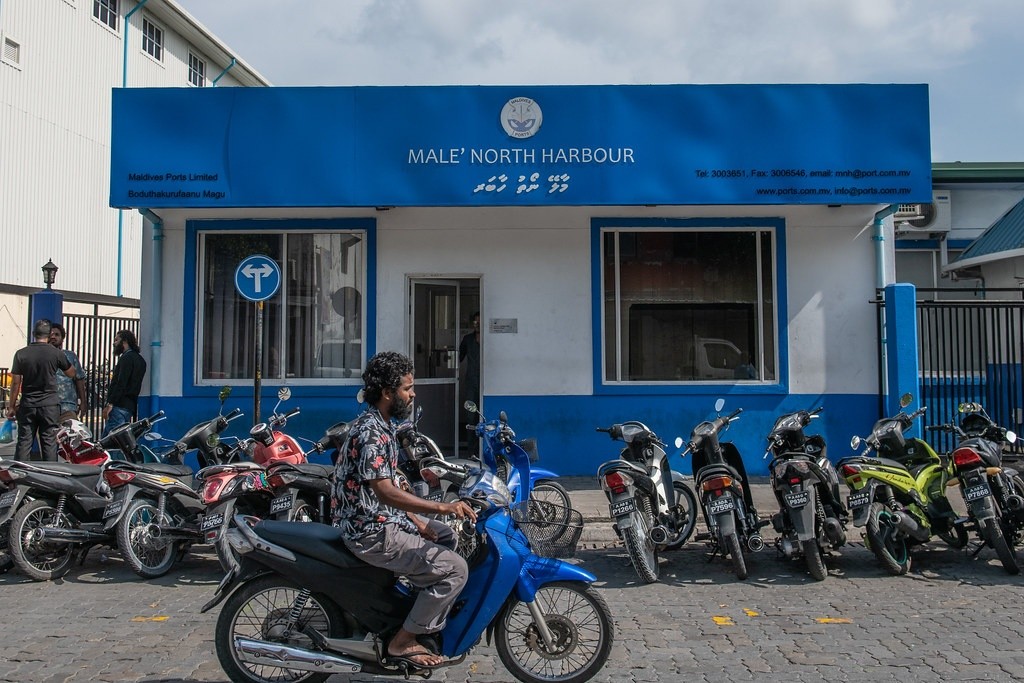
[261,342,292,378]
[30,323,88,461]
[7,319,76,462]
[99,330,147,460]
[443,311,480,462]
[734,351,759,380]
[764,355,775,379]
[330,351,479,670]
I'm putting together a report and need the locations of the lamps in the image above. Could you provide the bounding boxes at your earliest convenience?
[41,258,59,289]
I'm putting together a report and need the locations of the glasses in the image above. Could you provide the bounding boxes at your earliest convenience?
[472,320,479,328]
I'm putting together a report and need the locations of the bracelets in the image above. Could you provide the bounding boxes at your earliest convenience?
[106,402,113,408]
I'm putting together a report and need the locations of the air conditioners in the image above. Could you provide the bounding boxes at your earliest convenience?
[895,204,925,221]
[896,190,951,238]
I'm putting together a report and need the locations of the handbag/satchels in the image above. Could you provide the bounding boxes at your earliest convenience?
[0,417,14,443]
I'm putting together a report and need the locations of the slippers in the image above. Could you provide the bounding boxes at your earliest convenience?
[388,647,444,669]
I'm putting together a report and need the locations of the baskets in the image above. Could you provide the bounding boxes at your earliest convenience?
[516,438,539,462]
[511,499,583,558]
[151,445,185,466]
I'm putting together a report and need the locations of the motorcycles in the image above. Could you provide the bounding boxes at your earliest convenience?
[464,400,1024,573]
[203,462,614,682]
[1,386,571,577]
